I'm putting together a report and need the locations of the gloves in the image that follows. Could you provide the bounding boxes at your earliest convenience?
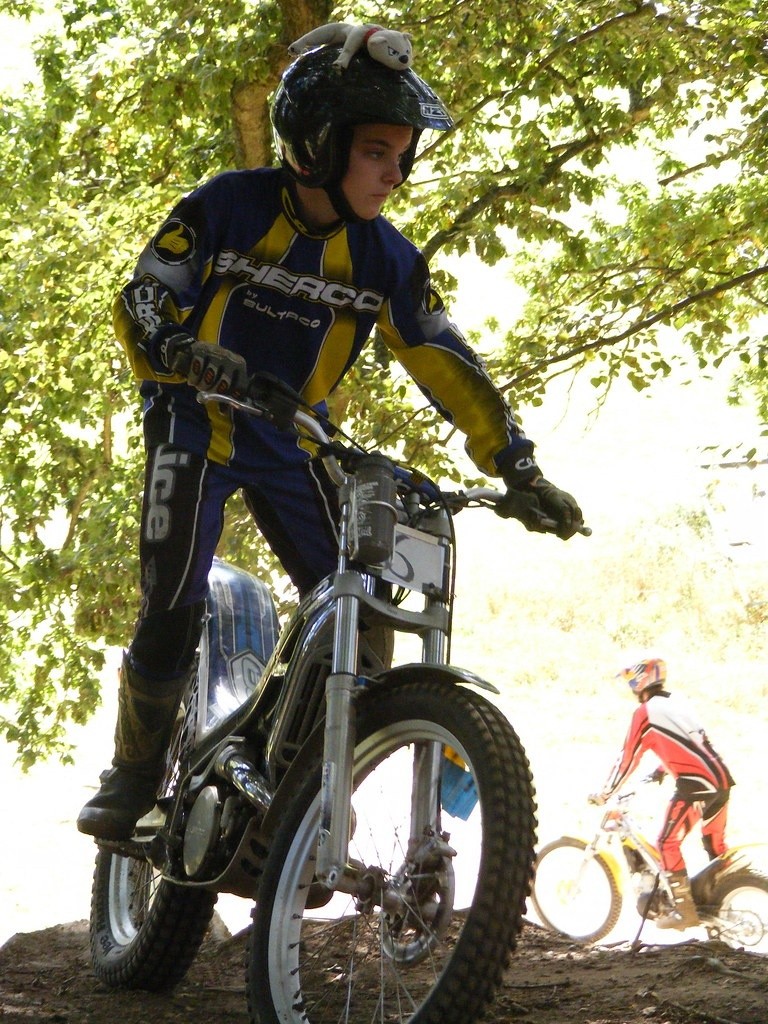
[644,769,665,785]
[159,332,248,414]
[587,794,606,806]
[495,439,584,541]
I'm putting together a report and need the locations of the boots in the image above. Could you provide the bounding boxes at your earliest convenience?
[656,870,699,930]
[298,798,355,910]
[78,649,191,841]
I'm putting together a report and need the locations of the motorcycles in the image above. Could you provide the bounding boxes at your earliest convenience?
[528,759,768,962]
[79,335,590,1024]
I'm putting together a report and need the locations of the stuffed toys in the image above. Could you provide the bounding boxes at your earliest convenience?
[286,23,416,76]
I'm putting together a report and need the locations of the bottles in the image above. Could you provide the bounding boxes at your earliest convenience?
[349,449,395,566]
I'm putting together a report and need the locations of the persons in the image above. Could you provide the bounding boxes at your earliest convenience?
[76,44,586,841]
[615,652,738,929]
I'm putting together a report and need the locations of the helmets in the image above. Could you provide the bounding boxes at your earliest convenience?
[270,43,453,190]
[615,658,666,694]
